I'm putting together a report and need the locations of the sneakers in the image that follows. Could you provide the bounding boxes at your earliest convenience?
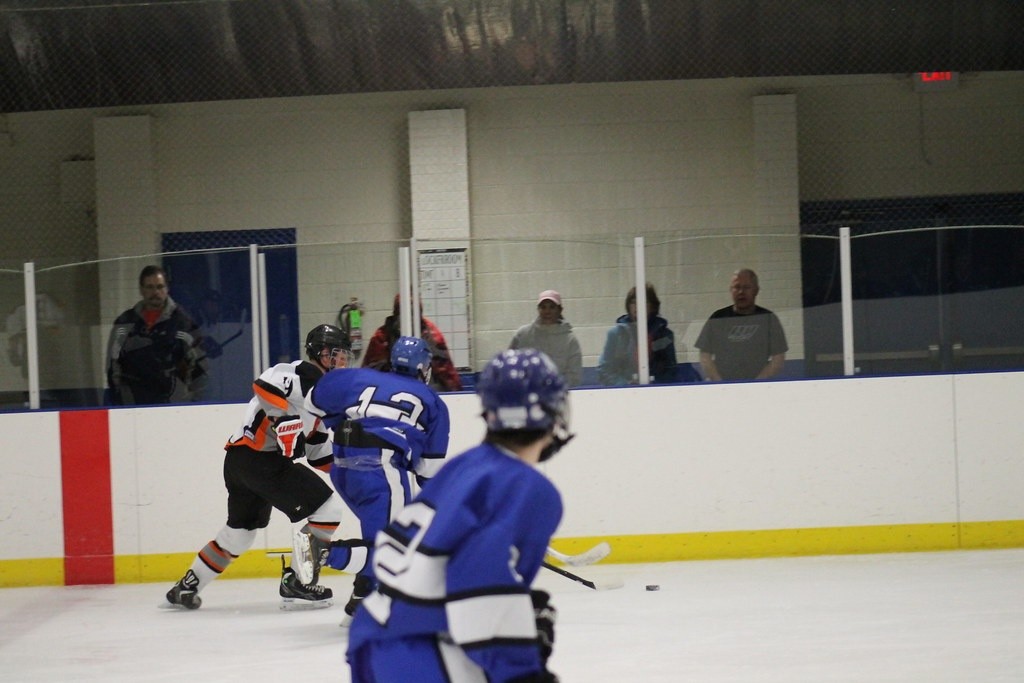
[340,573,376,626]
[279,554,333,611]
[164,569,202,609]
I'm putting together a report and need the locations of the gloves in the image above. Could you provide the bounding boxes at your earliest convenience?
[272,415,307,458]
[531,589,554,658]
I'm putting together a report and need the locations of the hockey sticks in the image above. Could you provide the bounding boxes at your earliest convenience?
[193,308,248,364]
[545,542,610,569]
[540,560,625,592]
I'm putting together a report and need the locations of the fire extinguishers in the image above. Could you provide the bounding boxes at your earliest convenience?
[339,297,367,352]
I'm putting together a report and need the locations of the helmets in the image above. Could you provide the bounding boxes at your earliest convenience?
[482,352,565,432]
[391,337,432,386]
[306,324,350,357]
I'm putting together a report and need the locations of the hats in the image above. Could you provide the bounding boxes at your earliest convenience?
[537,290,562,307]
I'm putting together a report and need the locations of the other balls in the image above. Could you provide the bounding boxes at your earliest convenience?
[645,585,660,592]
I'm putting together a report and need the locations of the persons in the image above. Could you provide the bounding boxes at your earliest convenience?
[106,265,208,406]
[158,324,352,610]
[302,336,450,628]
[694,269,788,382]
[509,291,582,391]
[346,350,569,683]
[598,286,680,385]
[361,294,463,392]
